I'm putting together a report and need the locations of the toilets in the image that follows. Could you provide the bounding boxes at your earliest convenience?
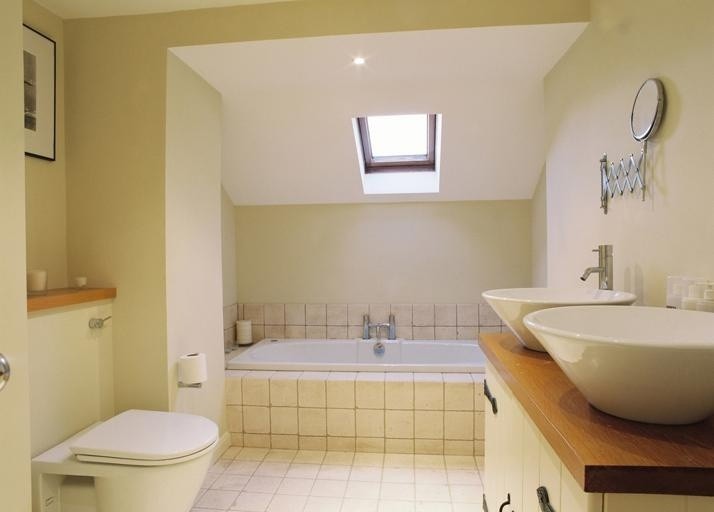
[34,408,219,512]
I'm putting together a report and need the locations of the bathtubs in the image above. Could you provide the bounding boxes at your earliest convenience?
[225,339,487,374]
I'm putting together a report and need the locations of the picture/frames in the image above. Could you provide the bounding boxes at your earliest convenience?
[23,22,56,161]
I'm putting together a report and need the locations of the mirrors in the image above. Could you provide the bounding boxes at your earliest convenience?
[630,78,665,202]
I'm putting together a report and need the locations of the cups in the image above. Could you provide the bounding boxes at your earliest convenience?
[28,270,48,297]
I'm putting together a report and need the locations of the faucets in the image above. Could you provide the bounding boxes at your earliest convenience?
[374,322,382,339]
[581,244,613,291]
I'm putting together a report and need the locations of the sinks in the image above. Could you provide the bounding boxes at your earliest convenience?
[481,288,636,350]
[522,304,714,425]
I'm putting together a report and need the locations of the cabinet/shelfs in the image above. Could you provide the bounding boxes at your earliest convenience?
[483,361,714,512]
